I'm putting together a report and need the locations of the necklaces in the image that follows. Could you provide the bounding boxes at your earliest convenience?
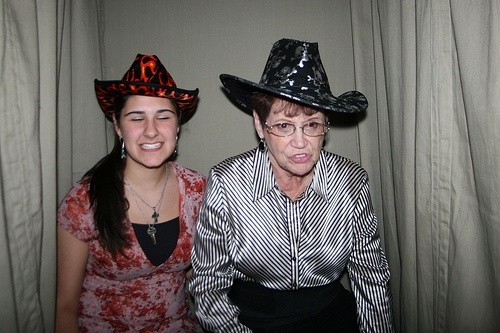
[127,185,165,245]
[122,163,170,224]
[290,173,310,204]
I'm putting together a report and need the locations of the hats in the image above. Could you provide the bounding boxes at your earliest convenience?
[219,37,369,116]
[93,53,199,126]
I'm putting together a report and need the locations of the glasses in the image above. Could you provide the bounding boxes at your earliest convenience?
[263,122,330,139]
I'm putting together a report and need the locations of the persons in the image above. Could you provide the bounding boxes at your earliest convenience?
[185,37,396,333]
[53,52,211,333]
